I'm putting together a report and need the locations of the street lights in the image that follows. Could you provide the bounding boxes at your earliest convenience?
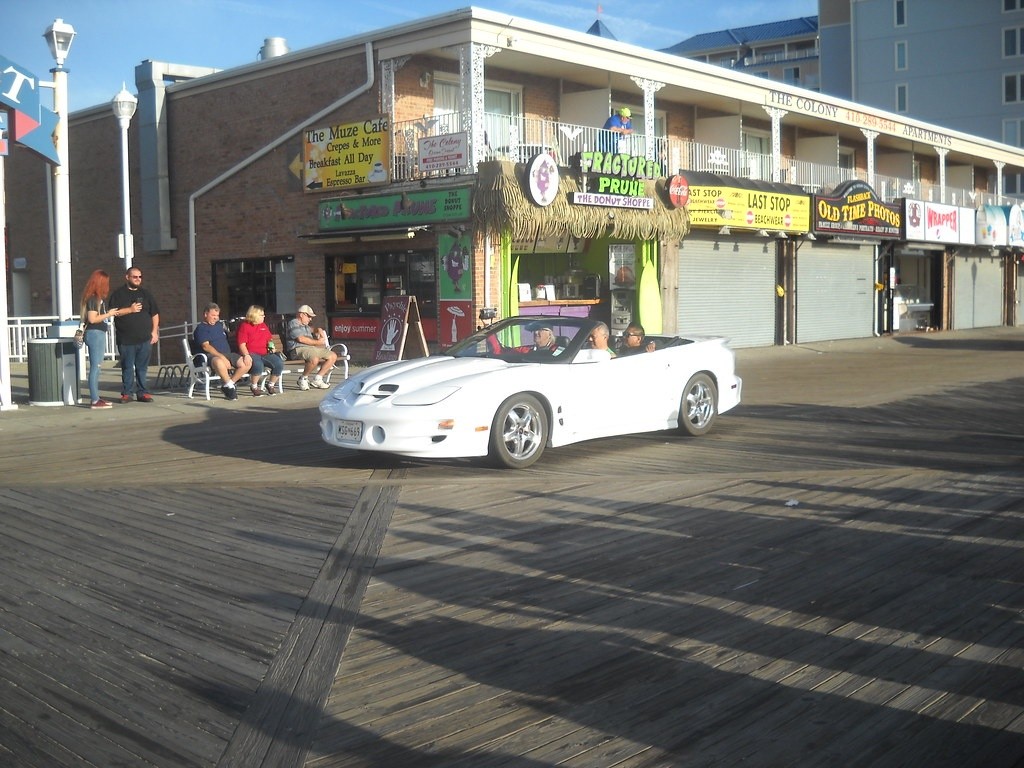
[110,79,140,284]
[42,16,85,407]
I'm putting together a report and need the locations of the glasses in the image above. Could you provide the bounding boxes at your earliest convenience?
[128,276,143,279]
[533,327,549,332]
[622,331,642,339]
[303,313,314,321]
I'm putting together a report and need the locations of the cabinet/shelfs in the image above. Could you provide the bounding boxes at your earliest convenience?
[215,261,276,314]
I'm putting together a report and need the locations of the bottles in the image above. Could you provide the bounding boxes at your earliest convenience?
[268,339,274,354]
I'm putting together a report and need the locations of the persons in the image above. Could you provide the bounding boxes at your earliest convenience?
[484,320,565,356]
[235,305,283,398]
[619,323,662,357]
[194,303,252,400]
[598,107,634,154]
[79,269,119,409]
[110,267,159,405]
[588,322,617,359]
[286,305,338,391]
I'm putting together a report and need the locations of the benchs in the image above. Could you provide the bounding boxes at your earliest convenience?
[183,333,350,400]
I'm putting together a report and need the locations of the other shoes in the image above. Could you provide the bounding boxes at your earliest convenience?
[265,381,276,394]
[228,388,238,401]
[137,393,153,402]
[91,400,112,409]
[99,398,113,405]
[220,386,230,398]
[120,394,133,403]
[250,385,261,396]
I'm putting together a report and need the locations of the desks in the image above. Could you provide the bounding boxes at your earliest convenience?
[499,144,556,163]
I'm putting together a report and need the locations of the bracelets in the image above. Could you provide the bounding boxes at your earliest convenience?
[244,354,250,356]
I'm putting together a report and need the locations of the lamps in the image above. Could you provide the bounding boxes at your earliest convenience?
[720,226,818,240]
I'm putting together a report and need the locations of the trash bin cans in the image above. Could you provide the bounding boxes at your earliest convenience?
[27,337,83,406]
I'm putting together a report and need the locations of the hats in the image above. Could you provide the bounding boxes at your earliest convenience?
[298,305,317,317]
[525,320,554,331]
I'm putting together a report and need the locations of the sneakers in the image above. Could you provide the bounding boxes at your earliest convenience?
[310,380,330,389]
[297,376,310,391]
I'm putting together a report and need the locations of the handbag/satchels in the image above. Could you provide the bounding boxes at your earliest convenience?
[73,329,83,349]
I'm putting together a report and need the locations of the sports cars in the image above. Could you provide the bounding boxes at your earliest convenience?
[317,312,745,470]
